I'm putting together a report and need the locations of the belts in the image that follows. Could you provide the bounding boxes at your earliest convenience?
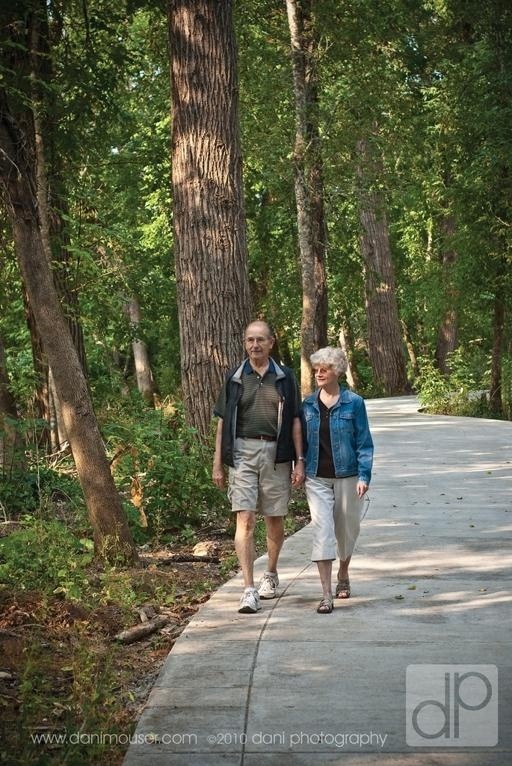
[237,433,276,442]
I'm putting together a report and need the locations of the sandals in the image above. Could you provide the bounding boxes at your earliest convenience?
[319,596,334,613]
[336,570,351,597]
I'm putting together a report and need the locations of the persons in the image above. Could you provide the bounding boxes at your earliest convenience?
[211,321,305,614]
[290,346,374,615]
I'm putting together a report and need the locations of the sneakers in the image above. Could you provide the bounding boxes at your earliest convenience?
[259,569,279,598]
[238,588,262,613]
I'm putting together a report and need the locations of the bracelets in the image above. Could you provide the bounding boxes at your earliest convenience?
[297,456,304,462]
